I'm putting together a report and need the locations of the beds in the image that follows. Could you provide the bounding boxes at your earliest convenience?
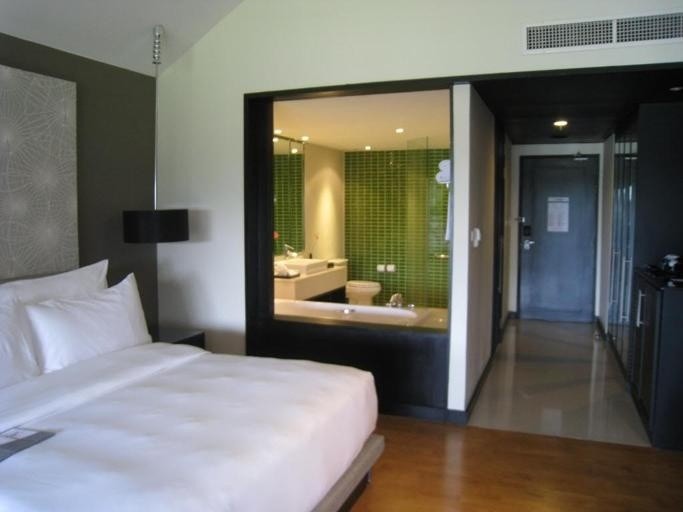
[0,339,386,512]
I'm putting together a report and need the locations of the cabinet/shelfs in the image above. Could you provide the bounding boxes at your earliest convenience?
[607,103,683,449]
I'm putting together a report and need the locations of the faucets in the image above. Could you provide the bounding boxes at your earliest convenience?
[389,293,403,308]
[285,244,297,258]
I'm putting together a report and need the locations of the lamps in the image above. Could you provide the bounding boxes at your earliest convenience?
[119,25,188,245]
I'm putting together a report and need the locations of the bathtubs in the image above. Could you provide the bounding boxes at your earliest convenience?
[276,300,415,318]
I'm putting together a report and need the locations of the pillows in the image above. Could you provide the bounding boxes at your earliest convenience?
[0,258,154,388]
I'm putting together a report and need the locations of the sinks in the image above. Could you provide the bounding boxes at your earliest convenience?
[283,259,328,273]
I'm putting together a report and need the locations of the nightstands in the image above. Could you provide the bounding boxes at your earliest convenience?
[151,327,205,349]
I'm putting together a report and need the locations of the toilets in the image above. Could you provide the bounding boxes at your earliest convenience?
[345,280,382,305]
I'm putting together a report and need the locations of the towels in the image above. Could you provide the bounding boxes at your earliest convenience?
[435,159,452,240]
